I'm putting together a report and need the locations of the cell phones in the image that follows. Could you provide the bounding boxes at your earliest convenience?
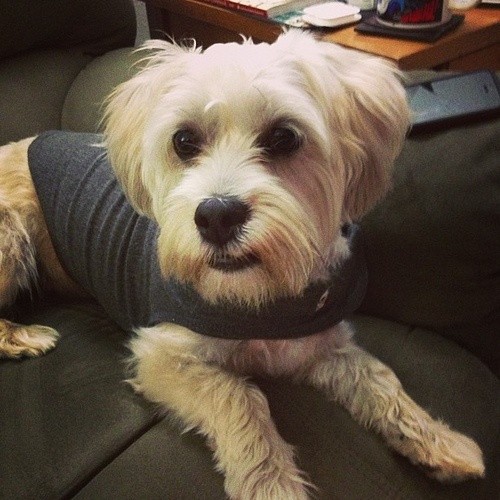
[404,67,500,135]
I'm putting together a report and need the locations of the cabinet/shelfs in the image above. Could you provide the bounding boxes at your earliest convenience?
[146,0,500,77]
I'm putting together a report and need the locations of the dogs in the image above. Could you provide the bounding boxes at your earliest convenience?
[0,27,485,500]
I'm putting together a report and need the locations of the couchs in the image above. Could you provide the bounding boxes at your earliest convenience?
[0,0,500,500]
